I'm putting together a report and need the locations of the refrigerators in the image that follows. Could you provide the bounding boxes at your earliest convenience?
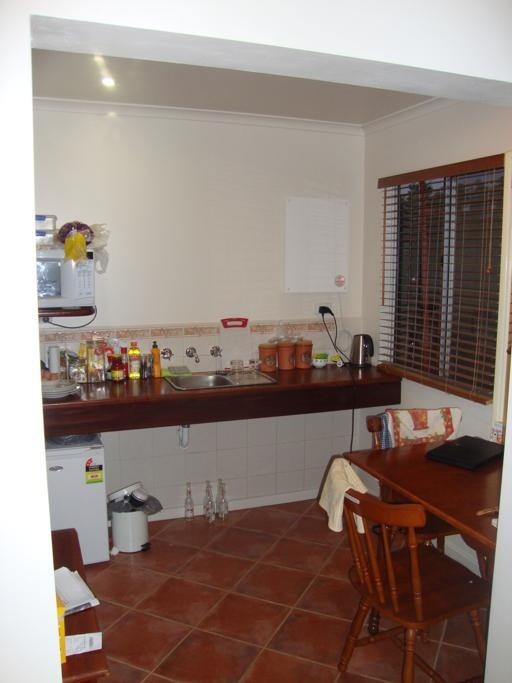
[46,434,112,566]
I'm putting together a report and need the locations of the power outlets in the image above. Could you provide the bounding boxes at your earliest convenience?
[312,303,336,318]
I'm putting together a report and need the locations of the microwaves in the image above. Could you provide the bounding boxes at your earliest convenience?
[36,250,96,310]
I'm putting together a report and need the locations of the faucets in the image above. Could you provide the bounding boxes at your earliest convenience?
[186,346,200,363]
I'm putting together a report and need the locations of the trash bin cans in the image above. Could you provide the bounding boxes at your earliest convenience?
[108,481,151,556]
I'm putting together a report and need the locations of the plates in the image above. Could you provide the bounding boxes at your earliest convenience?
[41,378,82,400]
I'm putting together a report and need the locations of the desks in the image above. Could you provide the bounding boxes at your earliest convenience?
[342,440,503,635]
[51,527,110,683]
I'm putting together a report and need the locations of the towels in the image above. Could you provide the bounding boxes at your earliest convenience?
[317,456,368,534]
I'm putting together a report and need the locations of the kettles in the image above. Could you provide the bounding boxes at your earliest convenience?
[349,333,375,369]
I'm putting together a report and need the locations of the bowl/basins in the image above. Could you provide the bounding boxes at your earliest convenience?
[312,353,328,369]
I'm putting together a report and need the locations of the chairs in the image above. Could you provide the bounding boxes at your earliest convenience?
[329,455,486,682]
[329,407,487,576]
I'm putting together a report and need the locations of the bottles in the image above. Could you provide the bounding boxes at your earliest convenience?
[276,339,295,371]
[184,481,195,522]
[257,342,278,373]
[203,478,229,528]
[121,340,162,382]
[294,336,313,370]
[59,334,108,385]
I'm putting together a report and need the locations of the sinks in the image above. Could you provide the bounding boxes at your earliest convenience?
[164,372,238,391]
